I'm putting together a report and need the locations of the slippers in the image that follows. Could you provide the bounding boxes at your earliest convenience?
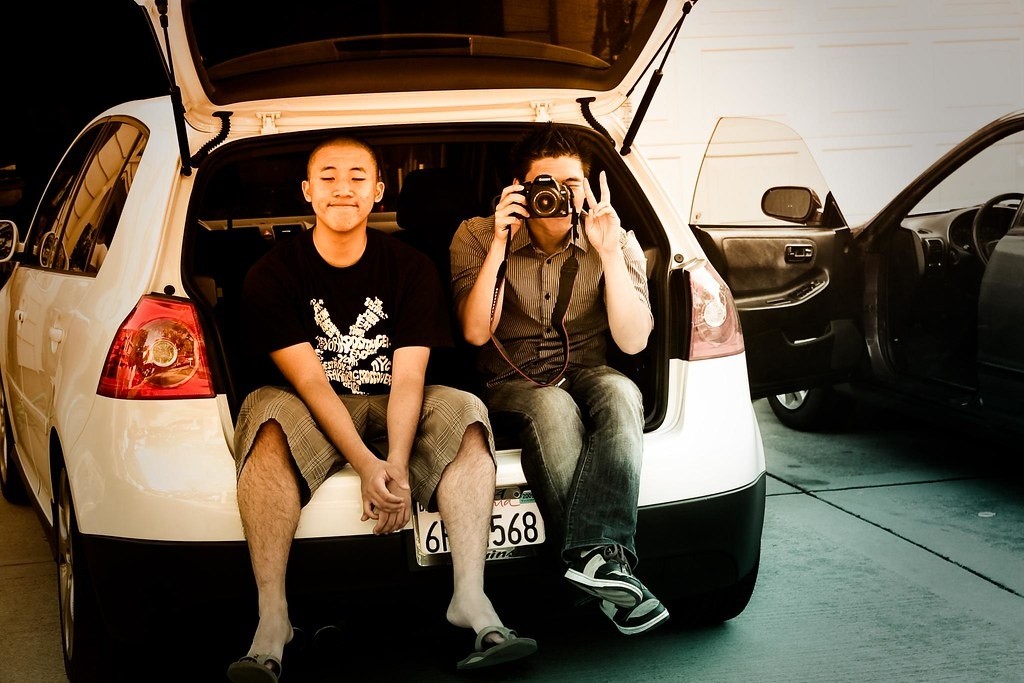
[456,626,538,671]
[227,655,283,683]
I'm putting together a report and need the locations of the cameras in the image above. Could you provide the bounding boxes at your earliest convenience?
[510,174,569,218]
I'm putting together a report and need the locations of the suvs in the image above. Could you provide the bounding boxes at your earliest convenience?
[1,1,772,683]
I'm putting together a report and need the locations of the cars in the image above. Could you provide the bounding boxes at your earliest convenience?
[689,100,1024,459]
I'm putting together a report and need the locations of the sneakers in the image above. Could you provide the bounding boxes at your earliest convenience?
[597,575,670,636]
[564,544,643,608]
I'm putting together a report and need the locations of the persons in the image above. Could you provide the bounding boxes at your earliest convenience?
[228,137,537,683]
[448,123,672,636]
